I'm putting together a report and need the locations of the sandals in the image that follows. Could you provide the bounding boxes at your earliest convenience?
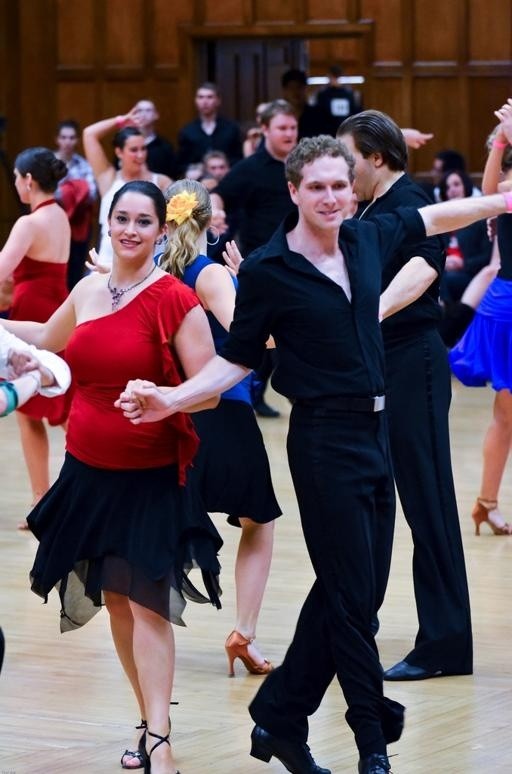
[139,731,183,774]
[122,714,172,769]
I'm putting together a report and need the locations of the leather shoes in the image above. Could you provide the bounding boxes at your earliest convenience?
[250,723,331,774]
[254,398,281,417]
[385,659,472,680]
[357,743,392,773]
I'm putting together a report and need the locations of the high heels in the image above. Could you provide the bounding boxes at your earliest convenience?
[225,632,275,677]
[473,496,512,535]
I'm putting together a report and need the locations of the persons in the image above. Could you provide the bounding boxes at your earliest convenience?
[1,65,511,774]
